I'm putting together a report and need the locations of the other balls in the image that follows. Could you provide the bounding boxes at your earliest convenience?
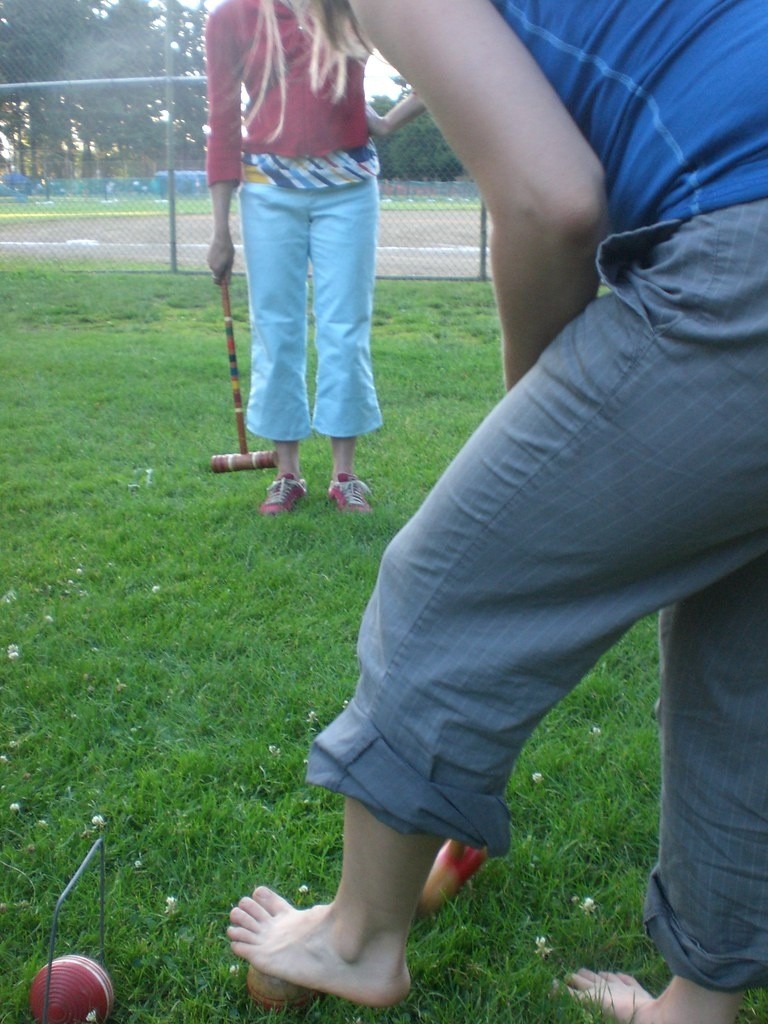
[29,953,116,1024]
[245,963,321,1013]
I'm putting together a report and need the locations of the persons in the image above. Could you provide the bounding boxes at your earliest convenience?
[227,0,768,1024]
[204,1,429,516]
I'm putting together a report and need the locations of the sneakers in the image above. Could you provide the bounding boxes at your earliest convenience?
[328,472,375,513]
[260,473,306,514]
